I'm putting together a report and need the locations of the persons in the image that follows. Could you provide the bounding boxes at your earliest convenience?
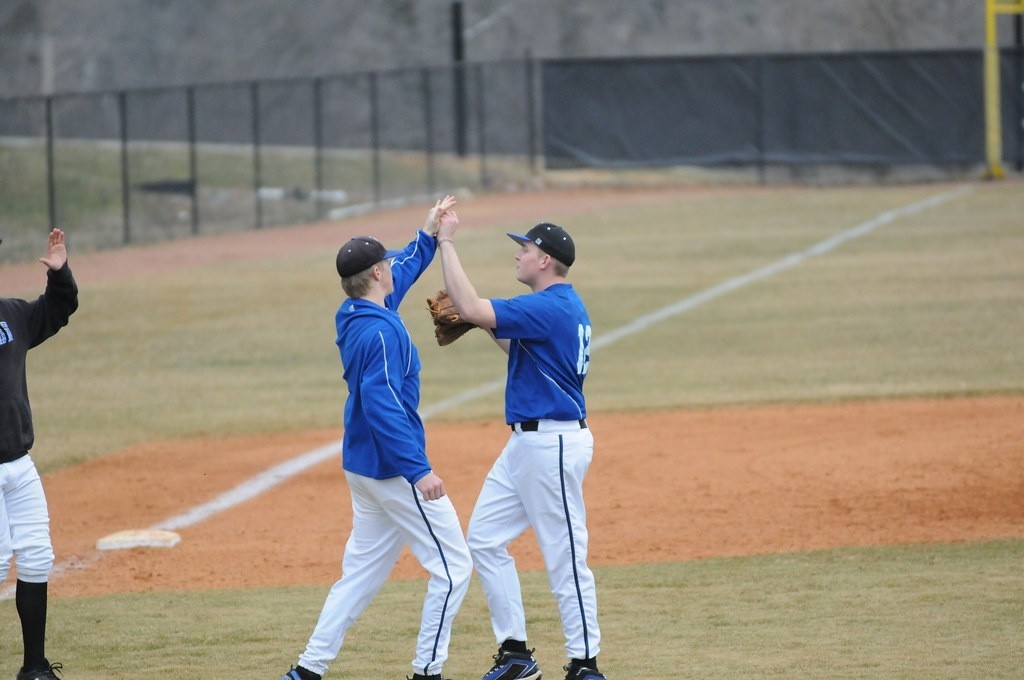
[280,196,474,680]
[0,229,79,680]
[436,211,607,680]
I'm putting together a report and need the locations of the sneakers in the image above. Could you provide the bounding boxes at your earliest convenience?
[482,647,541,679]
[565,660,603,680]
[16,659,62,679]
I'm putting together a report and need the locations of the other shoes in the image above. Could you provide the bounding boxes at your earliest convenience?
[282,666,300,680]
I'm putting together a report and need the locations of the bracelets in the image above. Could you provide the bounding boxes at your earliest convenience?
[438,239,454,247]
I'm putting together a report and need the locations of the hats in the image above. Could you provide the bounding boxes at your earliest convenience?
[507,223,576,269]
[336,238,406,278]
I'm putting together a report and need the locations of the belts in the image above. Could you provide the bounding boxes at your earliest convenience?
[511,417,587,433]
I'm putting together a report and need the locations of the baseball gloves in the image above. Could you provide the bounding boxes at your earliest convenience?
[425,288,479,347]
